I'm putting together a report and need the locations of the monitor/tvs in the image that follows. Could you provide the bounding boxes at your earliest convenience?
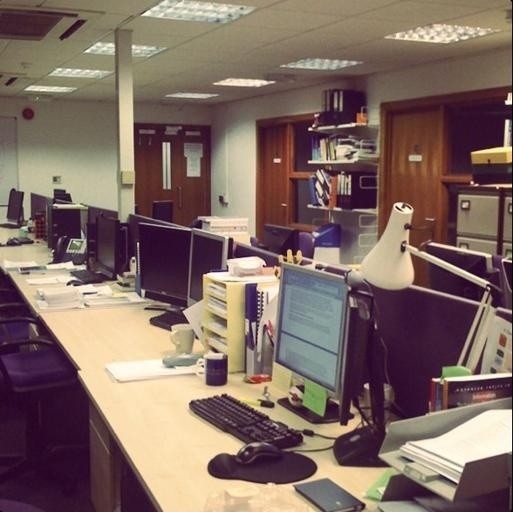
[275,262,355,424]
[189,228,233,303]
[427,239,504,309]
[7,191,26,227]
[95,216,129,280]
[334,297,394,468]
[264,225,299,271]
[139,223,191,309]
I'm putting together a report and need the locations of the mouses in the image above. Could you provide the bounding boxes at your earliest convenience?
[7,238,20,245]
[238,441,285,466]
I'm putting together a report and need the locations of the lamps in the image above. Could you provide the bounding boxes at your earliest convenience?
[358,199,504,375]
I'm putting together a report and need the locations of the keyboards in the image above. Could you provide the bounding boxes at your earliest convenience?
[70,269,102,283]
[150,311,201,340]
[0,222,20,230]
[189,394,303,450]
[13,237,35,244]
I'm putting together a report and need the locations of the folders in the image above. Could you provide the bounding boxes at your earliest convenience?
[336,171,377,209]
[323,88,366,125]
[310,169,330,206]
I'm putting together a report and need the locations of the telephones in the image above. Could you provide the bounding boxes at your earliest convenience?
[53,236,87,265]
[7,236,33,245]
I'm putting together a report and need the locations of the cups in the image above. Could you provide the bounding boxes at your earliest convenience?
[192,353,228,388]
[19,226,27,238]
[356,112,367,124]
[169,323,195,355]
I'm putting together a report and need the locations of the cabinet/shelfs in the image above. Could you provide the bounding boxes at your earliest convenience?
[441,175,512,260]
[305,122,380,214]
[201,270,279,373]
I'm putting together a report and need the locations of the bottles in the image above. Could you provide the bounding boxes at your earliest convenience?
[26,217,33,239]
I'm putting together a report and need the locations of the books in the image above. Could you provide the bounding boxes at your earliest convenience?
[430,373,512,409]
[296,477,365,512]
[319,133,376,161]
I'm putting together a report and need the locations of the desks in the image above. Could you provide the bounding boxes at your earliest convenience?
[8,265,144,315]
[37,300,206,369]
[0,221,55,274]
[76,354,406,512]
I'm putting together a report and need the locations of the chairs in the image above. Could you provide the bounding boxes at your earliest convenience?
[0,270,85,477]
